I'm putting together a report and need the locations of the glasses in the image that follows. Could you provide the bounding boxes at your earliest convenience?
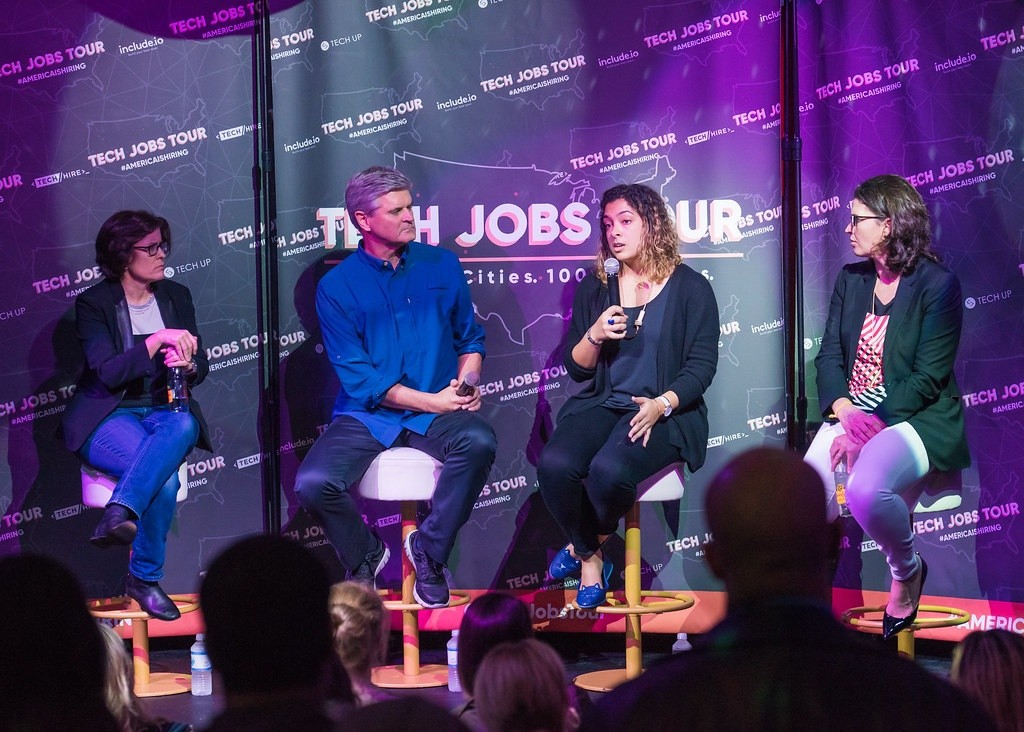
[850,214,882,227]
[133,241,169,256]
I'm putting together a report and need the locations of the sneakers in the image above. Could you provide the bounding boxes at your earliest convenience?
[405,530,451,607]
[345,527,391,590]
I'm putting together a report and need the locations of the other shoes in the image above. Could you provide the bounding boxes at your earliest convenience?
[577,562,612,609]
[550,545,579,580]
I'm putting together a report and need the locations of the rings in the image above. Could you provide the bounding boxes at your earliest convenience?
[608,317,614,325]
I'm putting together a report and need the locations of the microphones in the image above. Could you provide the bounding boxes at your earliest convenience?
[455,372,481,397]
[603,258,622,335]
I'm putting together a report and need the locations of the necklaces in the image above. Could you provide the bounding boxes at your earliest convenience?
[620,282,653,339]
[871,278,882,387]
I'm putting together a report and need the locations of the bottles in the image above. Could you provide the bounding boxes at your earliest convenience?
[191,634,212,696]
[834,453,852,517]
[672,633,692,654]
[167,366,189,413]
[447,630,463,692]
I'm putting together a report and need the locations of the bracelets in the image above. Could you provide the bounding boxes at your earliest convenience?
[587,329,602,348]
[828,400,851,419]
[654,398,661,413]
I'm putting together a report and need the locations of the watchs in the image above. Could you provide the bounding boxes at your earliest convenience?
[657,396,672,417]
[184,359,197,375]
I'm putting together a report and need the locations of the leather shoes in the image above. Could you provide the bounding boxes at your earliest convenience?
[89,504,137,550]
[126,573,181,620]
[878,552,927,637]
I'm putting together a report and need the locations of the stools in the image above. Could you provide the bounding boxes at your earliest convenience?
[572,462,696,693]
[841,467,971,662]
[81,460,200,699]
[354,445,471,689]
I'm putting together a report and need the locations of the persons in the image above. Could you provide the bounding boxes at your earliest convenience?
[802,173,972,641]
[536,183,721,610]
[0,440,1024,732]
[62,210,214,620]
[292,165,499,609]
